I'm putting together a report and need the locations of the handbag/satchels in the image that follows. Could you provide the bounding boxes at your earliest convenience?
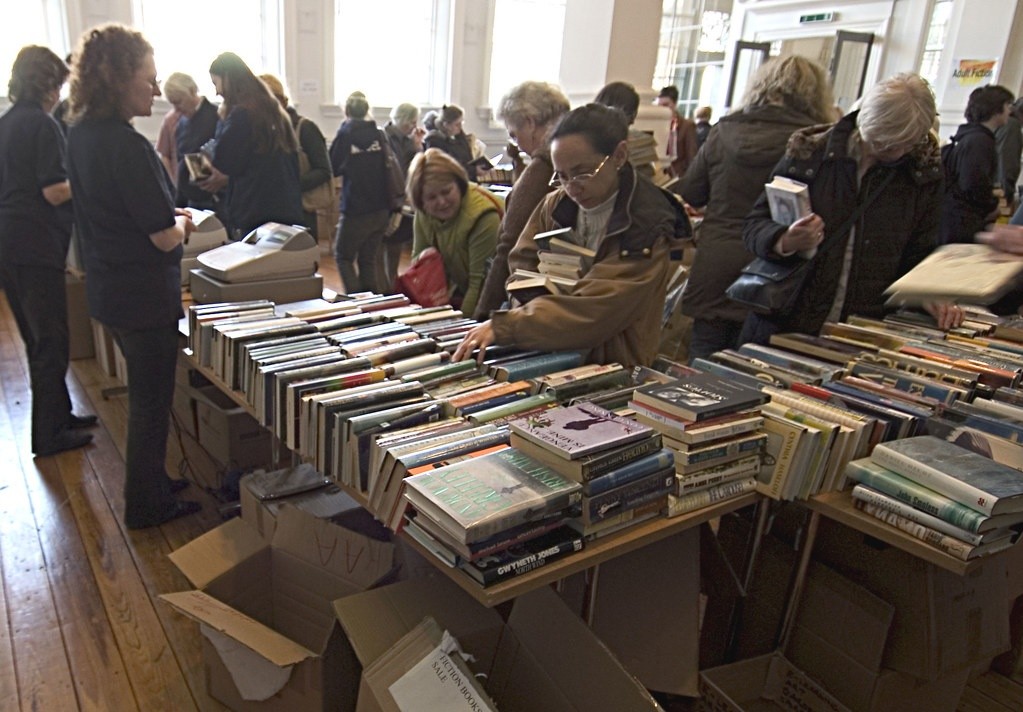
[294,118,336,212]
[721,257,813,316]
[379,127,406,210]
[395,248,449,307]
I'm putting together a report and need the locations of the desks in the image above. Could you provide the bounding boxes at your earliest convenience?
[185,356,759,636]
[777,488,988,660]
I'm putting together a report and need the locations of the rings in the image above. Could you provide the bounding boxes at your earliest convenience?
[954,305,960,309]
[817,232,822,237]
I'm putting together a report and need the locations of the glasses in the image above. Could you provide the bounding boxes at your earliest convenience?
[549,155,610,189]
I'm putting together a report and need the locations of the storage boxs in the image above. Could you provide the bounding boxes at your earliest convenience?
[840,519,1012,684]
[112,337,129,387]
[188,269,324,307]
[173,366,214,440]
[736,537,976,712]
[557,525,703,698]
[699,651,855,712]
[240,462,390,547]
[89,318,116,376]
[154,501,402,712]
[177,381,290,474]
[65,267,95,361]
[329,573,663,712]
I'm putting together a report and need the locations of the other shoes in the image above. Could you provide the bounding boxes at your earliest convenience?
[124,501,202,528]
[36,432,93,455]
[59,412,97,428]
[168,480,188,495]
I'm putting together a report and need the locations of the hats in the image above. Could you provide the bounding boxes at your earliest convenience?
[258,75,284,96]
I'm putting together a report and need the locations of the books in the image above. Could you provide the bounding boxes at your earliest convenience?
[506,227,597,305]
[187,290,1023,590]
[469,154,513,182]
[184,153,210,182]
[627,129,659,166]
[764,175,817,259]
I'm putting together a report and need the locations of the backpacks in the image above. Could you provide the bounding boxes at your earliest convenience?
[941,138,956,193]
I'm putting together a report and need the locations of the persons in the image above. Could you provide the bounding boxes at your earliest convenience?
[597,82,639,124]
[942,85,1023,253]
[739,72,966,346]
[155,72,220,210]
[0,46,98,454]
[260,75,489,295]
[597,501,618,514]
[654,387,708,401]
[472,81,572,323]
[679,54,838,370]
[659,86,712,177]
[760,452,775,466]
[452,103,695,367]
[198,52,304,239]
[405,148,507,318]
[63,24,200,530]
[779,200,792,224]
